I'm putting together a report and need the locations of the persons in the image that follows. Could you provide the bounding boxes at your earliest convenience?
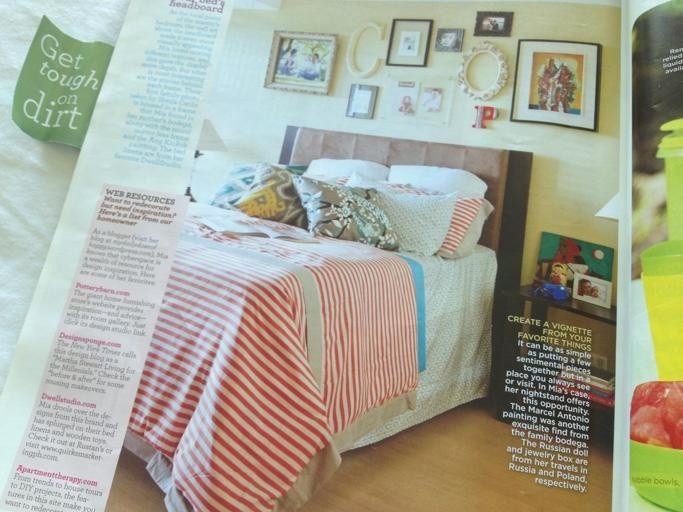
[280,49,297,75]
[440,32,456,47]
[398,96,414,113]
[577,279,601,298]
[298,53,320,80]
[423,89,442,112]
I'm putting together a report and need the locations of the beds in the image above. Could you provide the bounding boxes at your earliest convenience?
[117,123,537,512]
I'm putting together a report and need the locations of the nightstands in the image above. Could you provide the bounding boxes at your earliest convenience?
[502,282,615,431]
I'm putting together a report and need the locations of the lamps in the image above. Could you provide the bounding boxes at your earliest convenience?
[181,118,225,205]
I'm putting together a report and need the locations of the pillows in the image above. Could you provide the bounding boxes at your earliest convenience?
[204,155,497,262]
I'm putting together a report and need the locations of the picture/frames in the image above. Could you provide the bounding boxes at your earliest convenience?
[433,27,465,53]
[473,11,514,36]
[508,38,603,132]
[263,30,340,97]
[344,84,379,120]
[572,271,613,309]
[384,18,435,68]
